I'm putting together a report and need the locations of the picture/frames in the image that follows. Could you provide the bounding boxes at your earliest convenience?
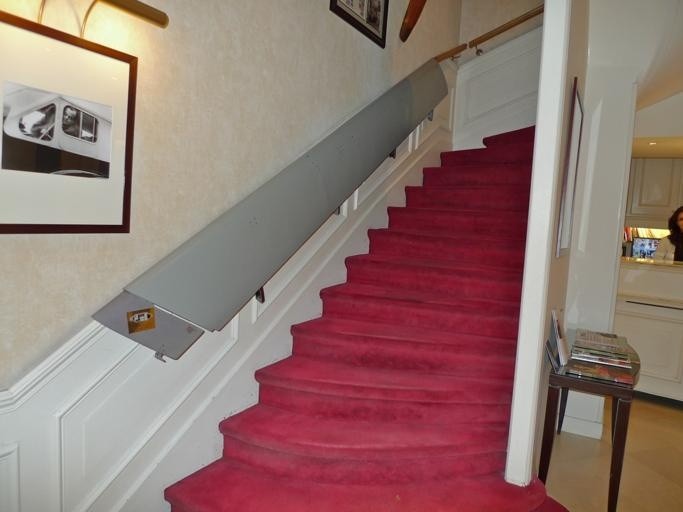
[555,76,584,260]
[328,0,390,50]
[1,10,140,235]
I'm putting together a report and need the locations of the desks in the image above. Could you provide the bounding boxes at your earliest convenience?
[537,347,635,512]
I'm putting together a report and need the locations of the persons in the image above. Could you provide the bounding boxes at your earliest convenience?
[45,105,80,140]
[651,206,683,262]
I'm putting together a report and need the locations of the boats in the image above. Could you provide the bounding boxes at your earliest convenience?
[129,312,153,323]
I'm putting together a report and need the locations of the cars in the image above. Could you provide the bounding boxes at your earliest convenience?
[2,82,111,177]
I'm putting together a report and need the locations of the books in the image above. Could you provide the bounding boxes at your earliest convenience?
[545,306,635,388]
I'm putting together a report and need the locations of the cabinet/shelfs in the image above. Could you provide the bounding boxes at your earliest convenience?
[609,157,682,407]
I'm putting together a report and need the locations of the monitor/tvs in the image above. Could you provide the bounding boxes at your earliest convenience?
[631,237,664,259]
